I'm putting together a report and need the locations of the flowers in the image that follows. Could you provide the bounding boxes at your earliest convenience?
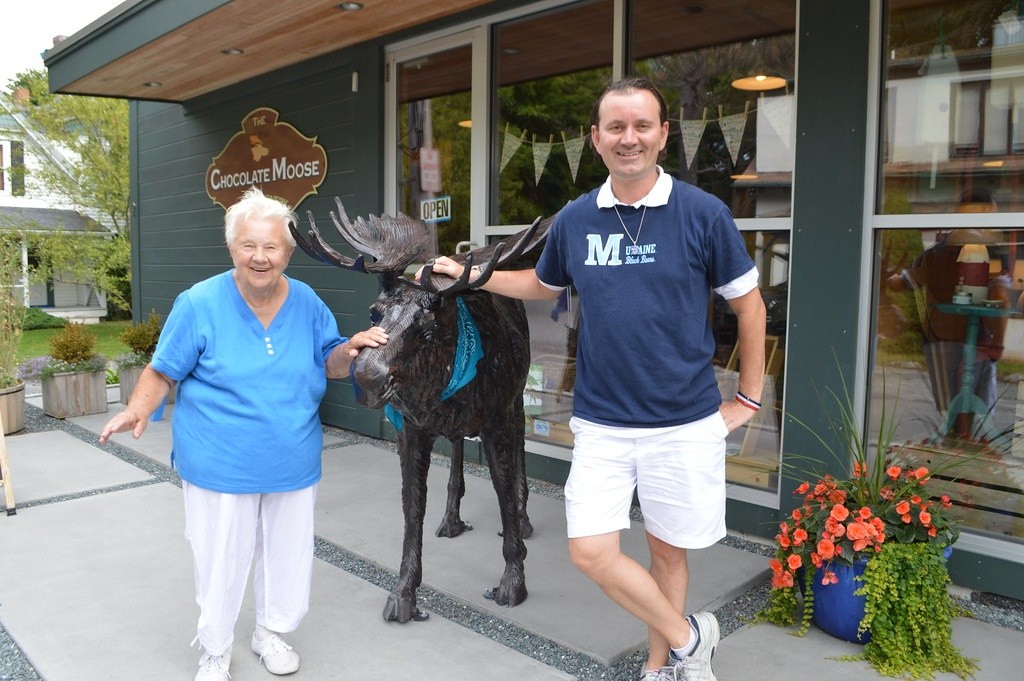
[733,346,983,681]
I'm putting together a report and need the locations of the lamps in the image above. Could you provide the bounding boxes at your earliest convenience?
[731,56,787,91]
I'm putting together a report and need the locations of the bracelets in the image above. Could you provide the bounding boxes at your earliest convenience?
[735,390,763,414]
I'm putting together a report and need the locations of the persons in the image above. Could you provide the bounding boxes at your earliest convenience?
[97,187,392,680]
[553,284,584,403]
[887,183,1012,445]
[415,74,767,681]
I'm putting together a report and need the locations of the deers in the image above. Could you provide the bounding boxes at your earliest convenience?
[287,197,571,624]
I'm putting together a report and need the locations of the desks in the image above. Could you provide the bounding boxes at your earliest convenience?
[928,300,1022,450]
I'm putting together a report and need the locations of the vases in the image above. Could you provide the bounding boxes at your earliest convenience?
[797,545,953,642]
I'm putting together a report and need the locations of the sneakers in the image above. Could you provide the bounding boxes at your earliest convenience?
[195,644,233,681]
[251,631,299,674]
[669,612,720,681]
[640,662,674,681]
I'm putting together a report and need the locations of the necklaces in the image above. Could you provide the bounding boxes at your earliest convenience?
[612,199,647,257]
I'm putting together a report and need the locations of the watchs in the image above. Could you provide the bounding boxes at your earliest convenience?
[468,264,488,291]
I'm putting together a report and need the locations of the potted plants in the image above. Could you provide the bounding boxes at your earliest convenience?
[0,236,35,435]
[39,317,108,419]
[118,307,174,406]
